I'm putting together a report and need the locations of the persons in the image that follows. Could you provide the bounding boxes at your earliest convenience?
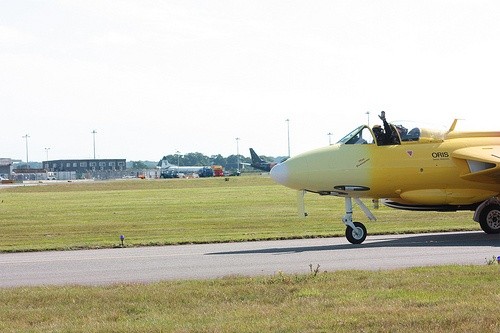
[372,109,394,146]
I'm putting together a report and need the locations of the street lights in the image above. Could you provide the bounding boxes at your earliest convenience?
[21,134,30,180]
[235,137,239,169]
[284,119,291,157]
[176,151,181,168]
[327,132,333,144]
[44,147,50,161]
[89,131,100,180]
[364,111,370,125]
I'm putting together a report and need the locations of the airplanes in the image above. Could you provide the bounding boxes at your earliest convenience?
[158,156,224,178]
[240,148,279,172]
[269,123,500,244]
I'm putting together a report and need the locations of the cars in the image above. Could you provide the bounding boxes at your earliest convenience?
[122,174,136,179]
[47,175,57,180]
